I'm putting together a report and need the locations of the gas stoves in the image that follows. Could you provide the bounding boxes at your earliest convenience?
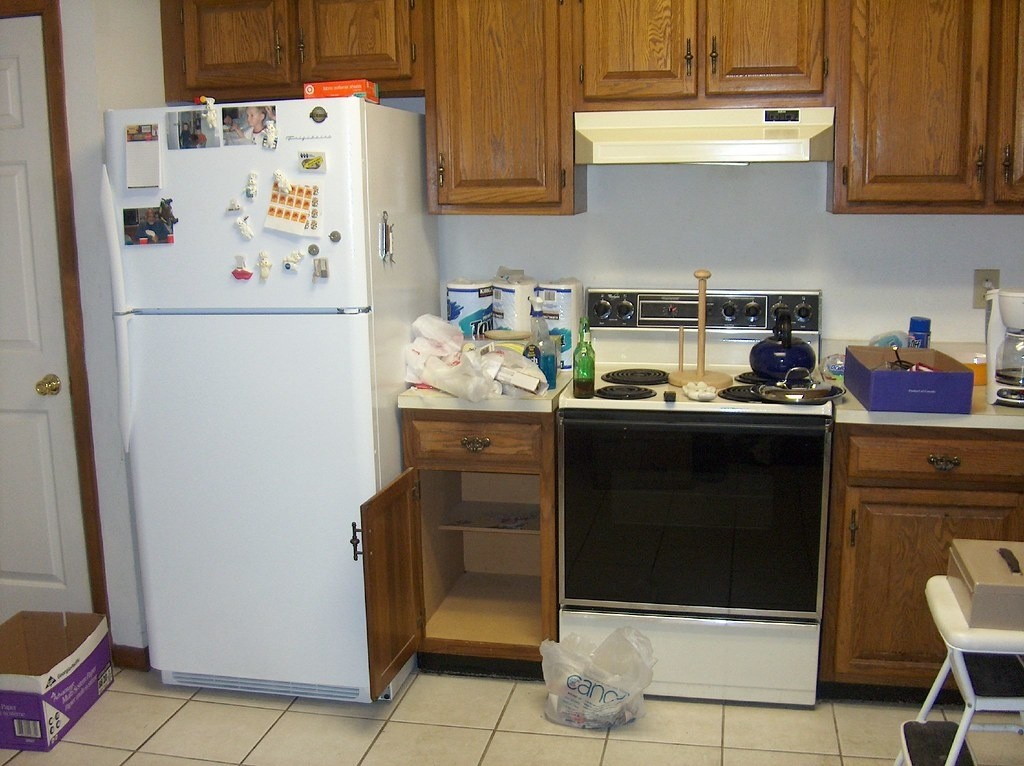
[560,287,833,416]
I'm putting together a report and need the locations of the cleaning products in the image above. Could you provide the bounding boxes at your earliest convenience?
[528,296,559,390]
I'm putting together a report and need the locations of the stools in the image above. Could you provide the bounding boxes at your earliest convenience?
[893,575,1024,766]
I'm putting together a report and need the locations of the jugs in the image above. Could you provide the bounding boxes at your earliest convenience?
[995,332,1024,386]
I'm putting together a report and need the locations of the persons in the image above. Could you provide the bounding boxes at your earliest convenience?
[223,106,278,146]
[179,123,207,149]
[133,208,170,241]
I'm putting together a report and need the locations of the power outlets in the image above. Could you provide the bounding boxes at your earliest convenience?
[973,269,1000,309]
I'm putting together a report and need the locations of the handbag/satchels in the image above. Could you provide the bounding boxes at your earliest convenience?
[539,627,656,728]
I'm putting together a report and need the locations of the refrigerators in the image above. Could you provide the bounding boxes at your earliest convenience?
[103,97,441,704]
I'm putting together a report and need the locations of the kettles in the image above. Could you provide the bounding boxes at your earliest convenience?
[750,308,816,380]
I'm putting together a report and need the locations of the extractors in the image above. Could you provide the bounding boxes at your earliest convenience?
[574,107,835,166]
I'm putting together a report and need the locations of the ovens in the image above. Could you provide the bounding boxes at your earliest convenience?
[556,408,834,705]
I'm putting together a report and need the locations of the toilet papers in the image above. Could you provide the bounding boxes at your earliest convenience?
[494,280,538,331]
[447,282,495,341]
[536,281,583,371]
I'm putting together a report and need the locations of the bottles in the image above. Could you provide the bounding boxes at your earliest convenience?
[908,316,932,348]
[572,316,595,399]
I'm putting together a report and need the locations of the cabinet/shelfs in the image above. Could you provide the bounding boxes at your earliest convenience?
[570,0,828,100]
[350,412,556,703]
[837,436,1023,690]
[842,0,1023,202]
[179,0,418,88]
[435,0,565,205]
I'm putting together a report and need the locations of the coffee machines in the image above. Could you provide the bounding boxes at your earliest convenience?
[984,287,1024,406]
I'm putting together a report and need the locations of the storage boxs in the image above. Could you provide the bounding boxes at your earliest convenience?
[0,610,115,751]
[844,344,974,415]
[303,78,380,104]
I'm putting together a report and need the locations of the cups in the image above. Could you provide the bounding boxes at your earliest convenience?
[958,351,986,385]
